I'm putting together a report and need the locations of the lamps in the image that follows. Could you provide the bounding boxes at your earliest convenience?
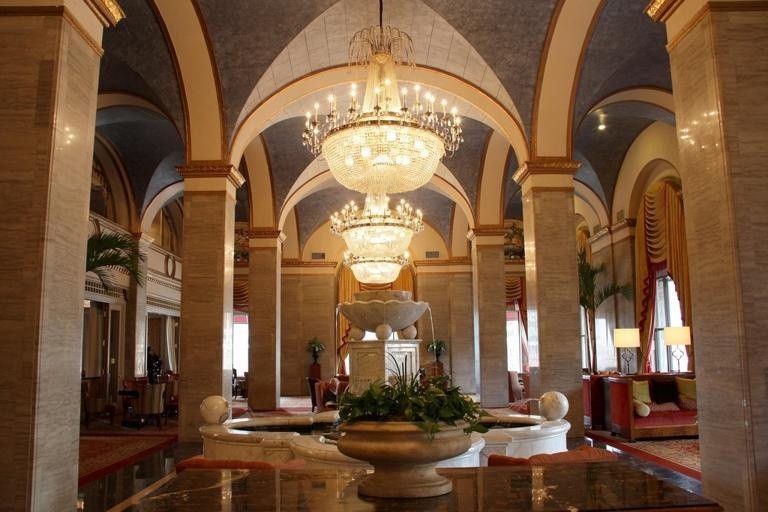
[663,326,692,373]
[613,328,640,374]
[299,2,464,285]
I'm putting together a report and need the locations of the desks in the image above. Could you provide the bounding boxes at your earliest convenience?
[117,458,724,511]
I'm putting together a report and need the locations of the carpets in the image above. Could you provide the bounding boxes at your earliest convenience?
[78,414,178,489]
[232,395,338,419]
[584,430,702,481]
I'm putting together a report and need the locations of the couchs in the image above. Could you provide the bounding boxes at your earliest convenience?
[606,373,699,443]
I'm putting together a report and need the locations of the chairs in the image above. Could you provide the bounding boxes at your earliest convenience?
[232,368,248,401]
[81,373,179,430]
[306,376,334,412]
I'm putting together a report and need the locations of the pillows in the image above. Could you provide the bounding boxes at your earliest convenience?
[631,375,697,417]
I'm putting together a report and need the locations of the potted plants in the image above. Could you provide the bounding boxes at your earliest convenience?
[426,338,449,362]
[332,348,497,499]
[304,336,328,363]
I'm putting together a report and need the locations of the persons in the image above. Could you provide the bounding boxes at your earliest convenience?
[324,377,340,408]
